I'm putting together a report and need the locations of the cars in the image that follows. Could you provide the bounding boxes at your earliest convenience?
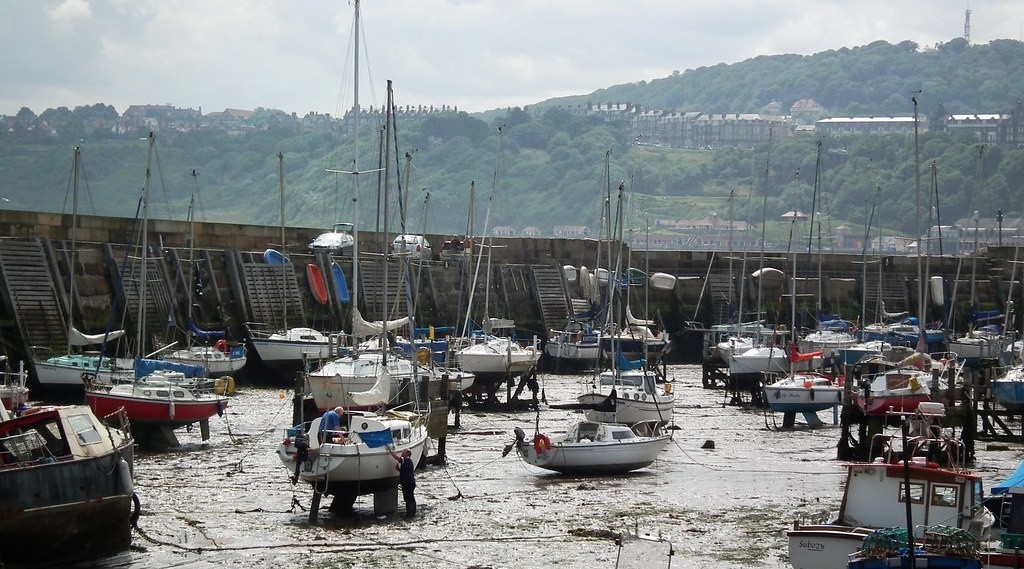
[438,240,471,262]
[307,232,355,257]
[389,233,434,260]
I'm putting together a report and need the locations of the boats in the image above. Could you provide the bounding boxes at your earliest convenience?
[1,1,1024,569]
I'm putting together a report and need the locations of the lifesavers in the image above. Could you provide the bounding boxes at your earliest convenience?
[415,347,431,366]
[21,405,57,416]
[216,340,229,354]
[280,437,299,462]
[535,434,551,454]
[215,376,236,395]
[456,337,471,350]
[897,459,939,470]
[634,393,647,401]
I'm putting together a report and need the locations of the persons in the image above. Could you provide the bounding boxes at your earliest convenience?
[318,406,345,445]
[386,445,417,520]
